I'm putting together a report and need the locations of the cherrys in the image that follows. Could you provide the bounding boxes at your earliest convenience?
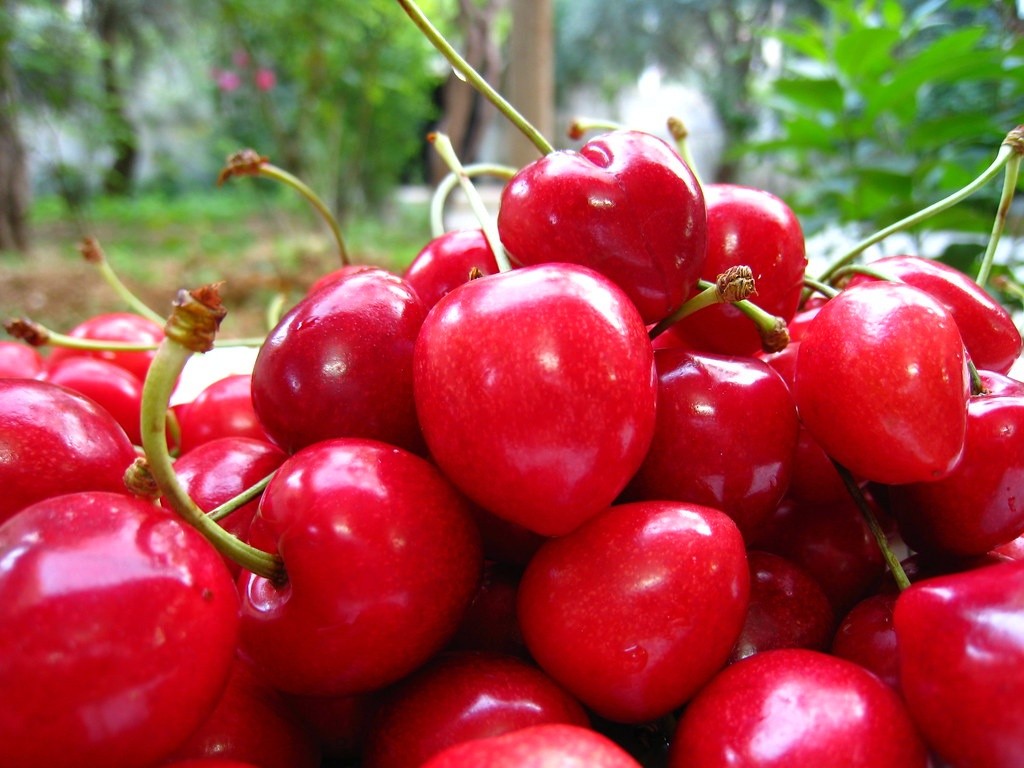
[3,0,1022,768]
